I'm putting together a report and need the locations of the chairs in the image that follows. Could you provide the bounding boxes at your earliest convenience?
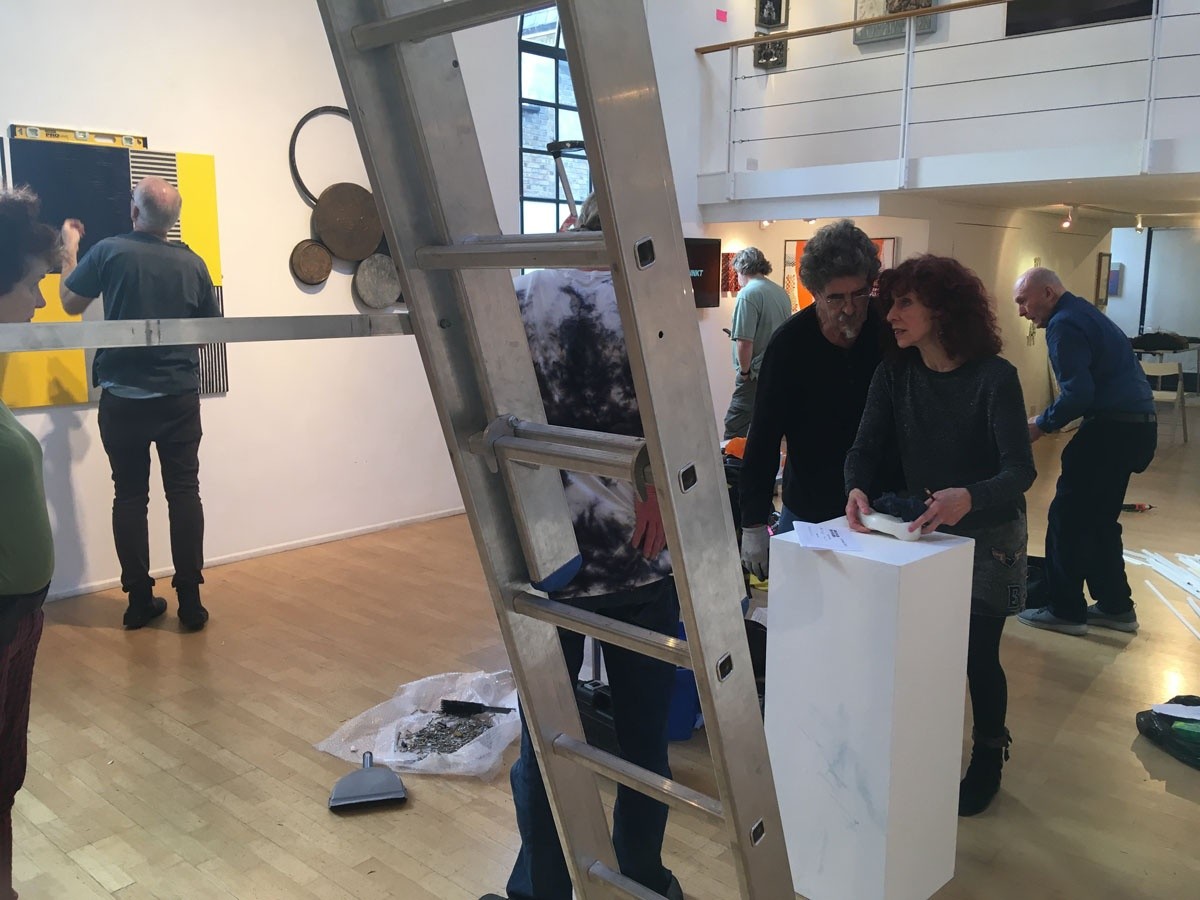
[1137,362,1188,448]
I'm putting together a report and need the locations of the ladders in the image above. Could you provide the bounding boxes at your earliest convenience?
[315,0,794,900]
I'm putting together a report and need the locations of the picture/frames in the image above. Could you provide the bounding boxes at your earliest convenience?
[784,237,898,316]
[853,0,936,45]
[1095,251,1112,308]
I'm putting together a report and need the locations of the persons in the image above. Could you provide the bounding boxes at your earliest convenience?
[505,194,688,900]
[843,251,1028,817]
[60,177,221,627]
[738,220,919,584]
[0,185,54,900]
[1013,267,1158,637]
[722,248,792,441]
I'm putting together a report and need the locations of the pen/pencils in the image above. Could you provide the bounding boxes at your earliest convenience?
[924,487,935,501]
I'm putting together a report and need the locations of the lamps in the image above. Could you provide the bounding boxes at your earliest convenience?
[760,220,776,230]
[1062,204,1079,230]
[1136,213,1144,234]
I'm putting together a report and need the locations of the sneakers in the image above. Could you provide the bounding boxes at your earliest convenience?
[1086,604,1140,633]
[1017,607,1089,636]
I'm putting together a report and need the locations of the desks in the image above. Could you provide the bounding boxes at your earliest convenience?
[1129,343,1200,415]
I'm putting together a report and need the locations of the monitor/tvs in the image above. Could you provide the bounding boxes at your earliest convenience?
[683,237,721,307]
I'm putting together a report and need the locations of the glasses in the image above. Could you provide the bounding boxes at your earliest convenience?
[815,289,872,308]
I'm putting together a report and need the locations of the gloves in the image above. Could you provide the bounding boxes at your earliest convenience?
[740,523,771,582]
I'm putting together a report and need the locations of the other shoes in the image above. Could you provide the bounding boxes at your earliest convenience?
[958,726,1012,817]
[124,595,167,631]
[177,604,210,632]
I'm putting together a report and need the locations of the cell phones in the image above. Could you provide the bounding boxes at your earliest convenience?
[723,328,731,334]
